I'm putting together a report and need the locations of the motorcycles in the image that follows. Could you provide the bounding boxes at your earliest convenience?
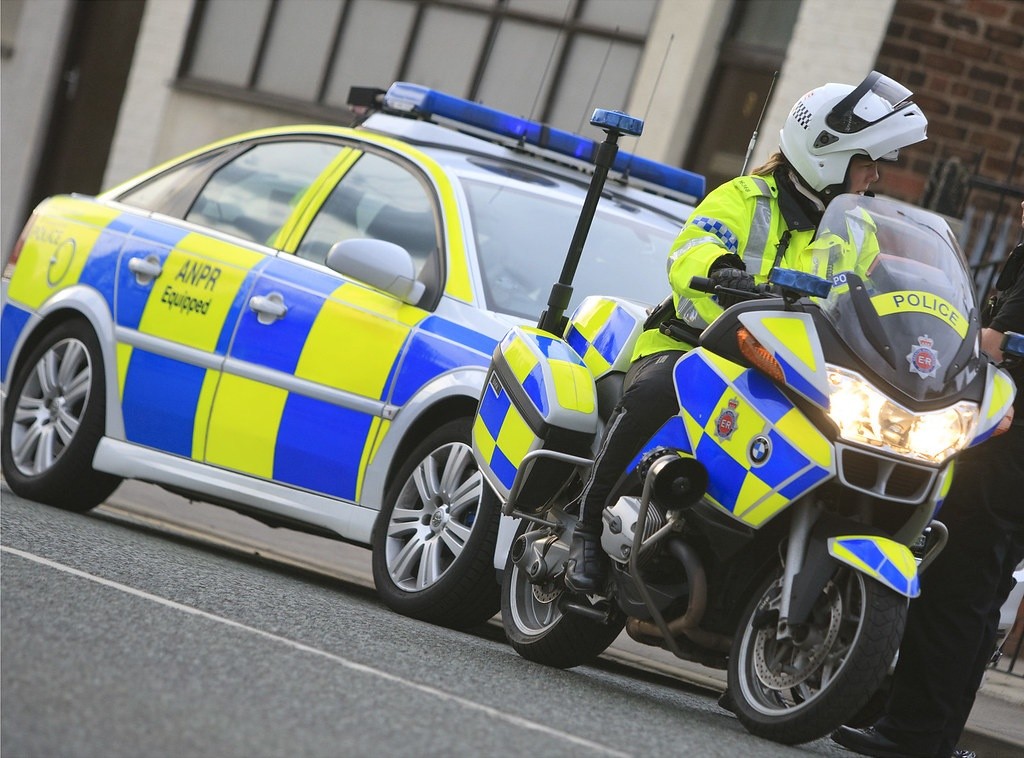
[470,194,1024,748]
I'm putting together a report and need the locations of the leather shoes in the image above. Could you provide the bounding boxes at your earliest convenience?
[564,523,605,595]
[831,723,918,758]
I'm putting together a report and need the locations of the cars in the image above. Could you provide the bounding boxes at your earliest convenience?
[0,82,1024,726]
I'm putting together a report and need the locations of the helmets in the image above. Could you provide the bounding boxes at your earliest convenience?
[778,70,928,192]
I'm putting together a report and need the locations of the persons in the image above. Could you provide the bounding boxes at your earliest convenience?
[562,68,928,594]
[832,199,1024,757]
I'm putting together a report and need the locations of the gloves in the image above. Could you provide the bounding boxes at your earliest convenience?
[709,266,756,310]
